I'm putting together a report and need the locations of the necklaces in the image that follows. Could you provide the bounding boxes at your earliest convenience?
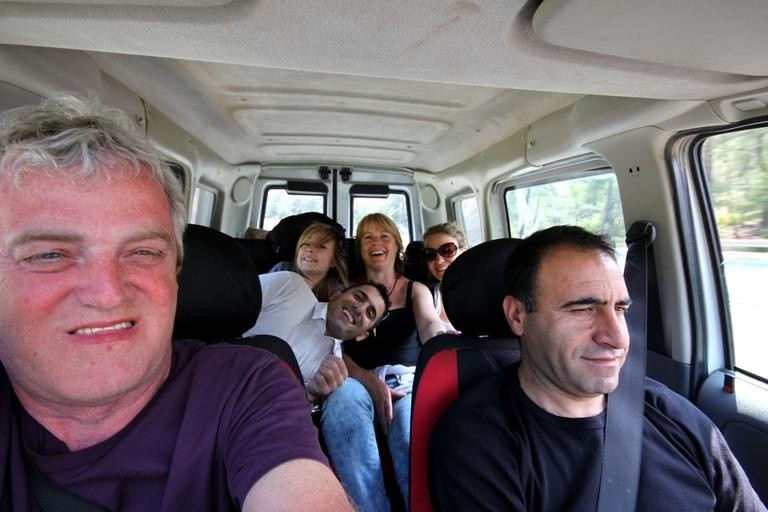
[388,279,397,297]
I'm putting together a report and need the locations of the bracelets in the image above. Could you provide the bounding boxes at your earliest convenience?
[310,404,321,413]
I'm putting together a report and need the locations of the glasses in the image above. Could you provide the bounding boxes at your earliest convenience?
[423,243,462,262]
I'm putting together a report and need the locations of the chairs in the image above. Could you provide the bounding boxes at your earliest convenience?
[403,237,533,509]
[168,226,308,396]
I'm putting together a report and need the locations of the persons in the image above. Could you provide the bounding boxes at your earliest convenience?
[0,94,355,511]
[268,222,348,301]
[429,224,767,511]
[240,270,393,414]
[321,213,463,512]
[420,222,486,336]
